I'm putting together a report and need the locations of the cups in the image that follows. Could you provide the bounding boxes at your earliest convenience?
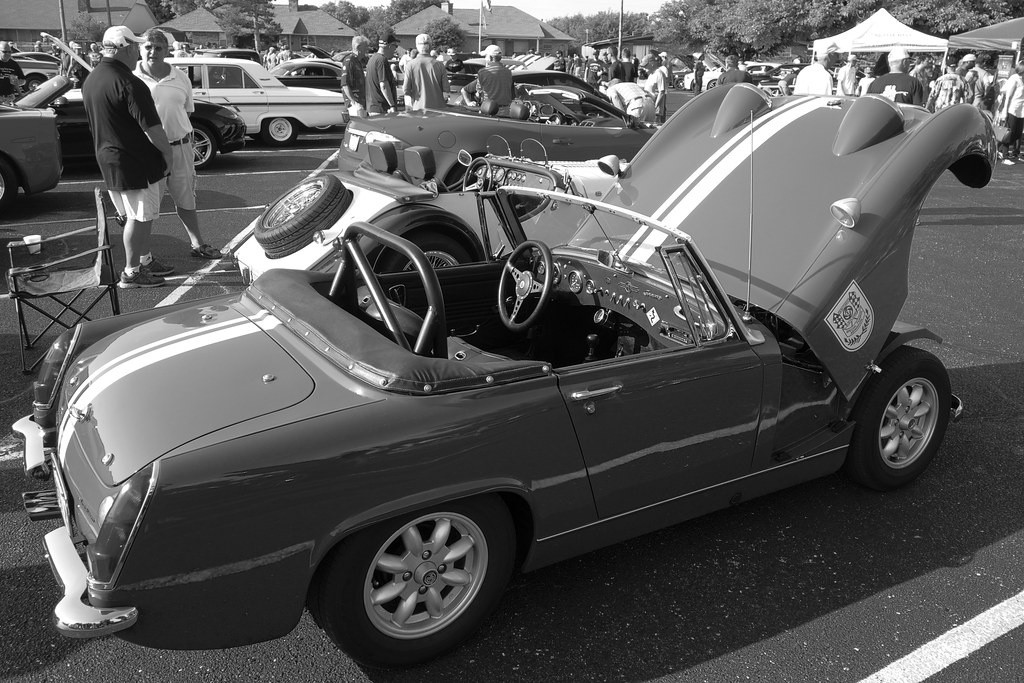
[23,235,41,254]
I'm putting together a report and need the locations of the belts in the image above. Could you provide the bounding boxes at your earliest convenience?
[635,94,650,100]
[170,137,190,145]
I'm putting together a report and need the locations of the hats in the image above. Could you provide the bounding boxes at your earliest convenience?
[640,54,655,67]
[847,54,860,62]
[946,55,959,67]
[658,52,667,58]
[963,54,976,62]
[817,40,840,54]
[696,53,704,60]
[864,68,873,73]
[888,48,917,62]
[102,25,147,49]
[378,32,401,45]
[72,43,82,48]
[479,44,503,57]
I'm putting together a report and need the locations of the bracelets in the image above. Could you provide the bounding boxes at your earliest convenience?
[390,105,396,107]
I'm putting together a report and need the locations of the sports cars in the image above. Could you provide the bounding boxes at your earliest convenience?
[3,75,247,170]
[336,84,661,194]
[8,81,999,671]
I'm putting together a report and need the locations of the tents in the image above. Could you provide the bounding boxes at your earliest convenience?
[811,8,948,71]
[943,17,1024,75]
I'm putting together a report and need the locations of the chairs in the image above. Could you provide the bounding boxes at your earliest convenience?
[509,103,532,122]
[481,98,499,118]
[7,187,122,375]
[349,283,513,365]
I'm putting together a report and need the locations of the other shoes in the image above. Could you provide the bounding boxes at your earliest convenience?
[1001,159,1015,165]
[1008,153,1015,158]
[1018,155,1024,162]
[996,150,1004,159]
[190,243,223,260]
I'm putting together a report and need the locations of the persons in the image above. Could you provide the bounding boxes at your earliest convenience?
[994,60,1024,165]
[0,41,28,96]
[52,40,103,90]
[926,54,993,114]
[461,45,515,114]
[174,41,239,75]
[717,55,752,84]
[526,44,673,127]
[331,48,335,56]
[909,55,942,107]
[778,51,888,97]
[35,41,43,51]
[398,34,464,110]
[366,34,400,116]
[341,36,370,111]
[512,52,517,60]
[867,49,922,106]
[694,55,705,93]
[131,30,222,260]
[84,26,175,288]
[263,44,294,69]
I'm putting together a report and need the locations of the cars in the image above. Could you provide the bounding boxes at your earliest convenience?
[636,47,874,98]
[60,56,352,147]
[8,51,72,99]
[189,45,612,103]
[0,101,64,210]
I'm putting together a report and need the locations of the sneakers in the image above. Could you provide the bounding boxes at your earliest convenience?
[118,264,168,288]
[142,257,176,276]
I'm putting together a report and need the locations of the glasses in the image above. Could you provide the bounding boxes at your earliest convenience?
[1,50,13,54]
[142,45,167,52]
[389,44,398,48]
